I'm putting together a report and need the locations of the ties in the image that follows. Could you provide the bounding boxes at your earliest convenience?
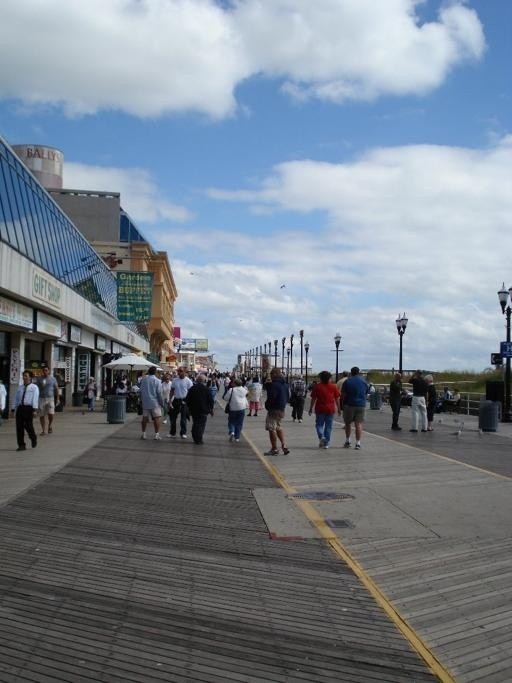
[21,385,27,406]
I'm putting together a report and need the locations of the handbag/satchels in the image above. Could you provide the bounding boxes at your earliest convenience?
[138,398,144,416]
[225,404,231,414]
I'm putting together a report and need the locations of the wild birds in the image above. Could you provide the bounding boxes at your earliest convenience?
[478,427,484,437]
[438,417,442,424]
[454,418,460,423]
[189,272,194,275]
[448,429,462,440]
[280,284,286,289]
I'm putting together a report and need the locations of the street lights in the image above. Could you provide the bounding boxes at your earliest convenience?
[211,352,216,371]
[330,327,344,383]
[189,362,193,371]
[243,329,310,387]
[193,348,199,369]
[395,310,408,393]
[496,278,512,422]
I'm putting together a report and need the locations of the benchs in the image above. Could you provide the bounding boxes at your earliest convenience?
[439,396,462,415]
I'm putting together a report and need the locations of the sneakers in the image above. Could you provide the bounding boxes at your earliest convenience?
[409,428,434,432]
[39,429,52,435]
[195,441,204,444]
[166,434,176,439]
[319,437,325,447]
[247,414,258,416]
[391,426,402,431]
[283,447,291,455]
[325,446,329,449]
[355,445,361,450]
[229,432,239,442]
[264,448,279,456]
[293,419,303,423]
[141,434,147,440]
[344,442,352,449]
[182,435,188,439]
[154,436,162,440]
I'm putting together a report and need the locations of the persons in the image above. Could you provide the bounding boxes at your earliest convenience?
[36,363,63,436]
[137,365,165,440]
[185,372,216,445]
[262,367,292,457]
[307,370,343,451]
[223,376,251,443]
[11,368,40,453]
[165,365,193,440]
[341,367,369,449]
[0,377,7,415]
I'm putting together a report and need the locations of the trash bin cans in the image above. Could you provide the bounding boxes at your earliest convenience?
[210,387,217,397]
[107,395,126,424]
[73,391,84,406]
[370,393,382,409]
[478,401,499,432]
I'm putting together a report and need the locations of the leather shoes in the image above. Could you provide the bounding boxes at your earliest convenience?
[31,437,37,448]
[15,446,27,451]
